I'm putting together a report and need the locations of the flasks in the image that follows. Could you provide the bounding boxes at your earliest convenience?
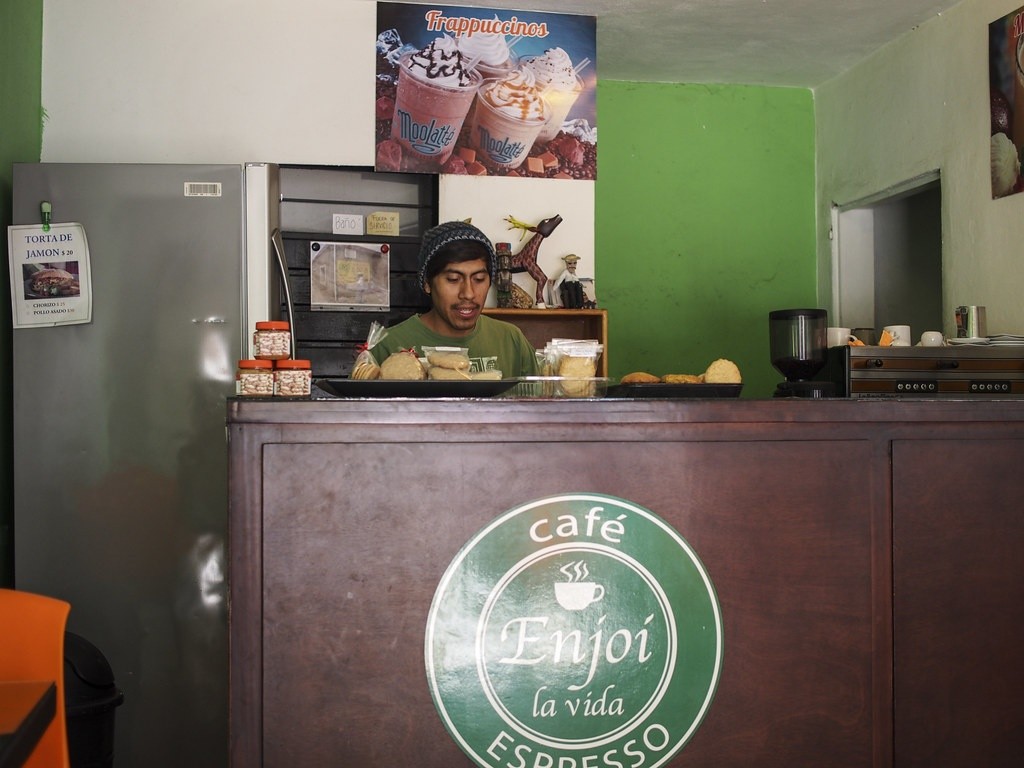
[967,305,979,337]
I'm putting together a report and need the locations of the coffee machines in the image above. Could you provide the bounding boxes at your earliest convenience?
[768,308,829,398]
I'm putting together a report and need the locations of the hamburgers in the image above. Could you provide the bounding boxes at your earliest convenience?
[29,269,74,296]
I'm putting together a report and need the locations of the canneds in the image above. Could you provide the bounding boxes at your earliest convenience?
[236,321,311,397]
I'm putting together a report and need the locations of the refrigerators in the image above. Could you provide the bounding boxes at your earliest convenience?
[9,159,246,768]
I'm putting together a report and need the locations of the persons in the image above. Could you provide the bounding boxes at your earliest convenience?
[551,255,594,309]
[369,221,542,380]
[496,256,513,307]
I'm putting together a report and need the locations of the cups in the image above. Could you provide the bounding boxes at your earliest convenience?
[451,32,520,126]
[468,76,552,174]
[387,50,484,165]
[882,324,911,347]
[920,330,946,347]
[825,326,859,349]
[515,53,586,144]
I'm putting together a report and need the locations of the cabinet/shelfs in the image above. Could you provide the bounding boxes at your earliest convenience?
[275,162,441,406]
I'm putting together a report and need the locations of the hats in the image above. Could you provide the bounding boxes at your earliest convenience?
[418,221,497,296]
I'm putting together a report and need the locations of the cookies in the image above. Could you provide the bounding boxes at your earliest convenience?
[621,357,742,384]
[351,352,503,380]
[543,356,597,398]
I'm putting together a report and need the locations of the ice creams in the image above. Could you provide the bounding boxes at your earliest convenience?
[404,14,580,124]
